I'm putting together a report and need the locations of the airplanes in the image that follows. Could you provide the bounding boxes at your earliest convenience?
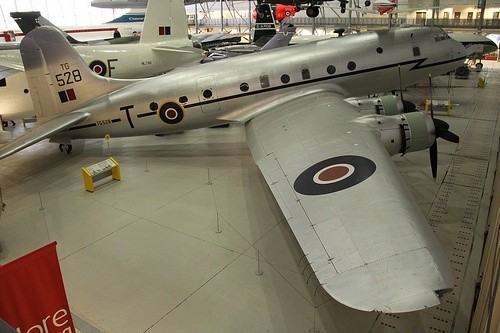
[249,1,398,21]
[443,30,497,78]
[0,6,147,50]
[3,24,470,313]
[0,1,212,130]
[11,12,203,49]
